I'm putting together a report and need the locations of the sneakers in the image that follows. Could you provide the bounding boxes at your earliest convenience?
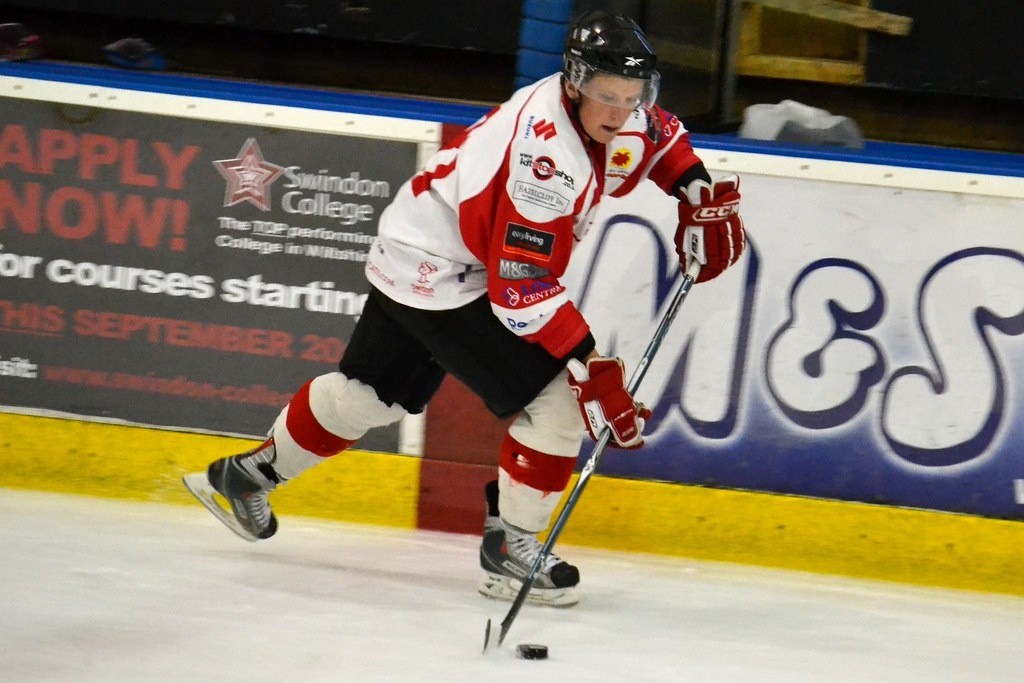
[183,437,290,542]
[479,480,582,606]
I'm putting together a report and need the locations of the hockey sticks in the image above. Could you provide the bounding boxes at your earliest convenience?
[480,257,702,656]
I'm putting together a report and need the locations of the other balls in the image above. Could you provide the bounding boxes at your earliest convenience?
[516,643,548,660]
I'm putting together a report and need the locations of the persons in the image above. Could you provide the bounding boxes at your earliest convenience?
[181,6,748,606]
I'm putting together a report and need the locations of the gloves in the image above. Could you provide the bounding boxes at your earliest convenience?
[674,172,748,283]
[566,355,652,450]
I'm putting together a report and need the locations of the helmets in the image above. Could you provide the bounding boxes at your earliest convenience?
[562,7,658,91]
